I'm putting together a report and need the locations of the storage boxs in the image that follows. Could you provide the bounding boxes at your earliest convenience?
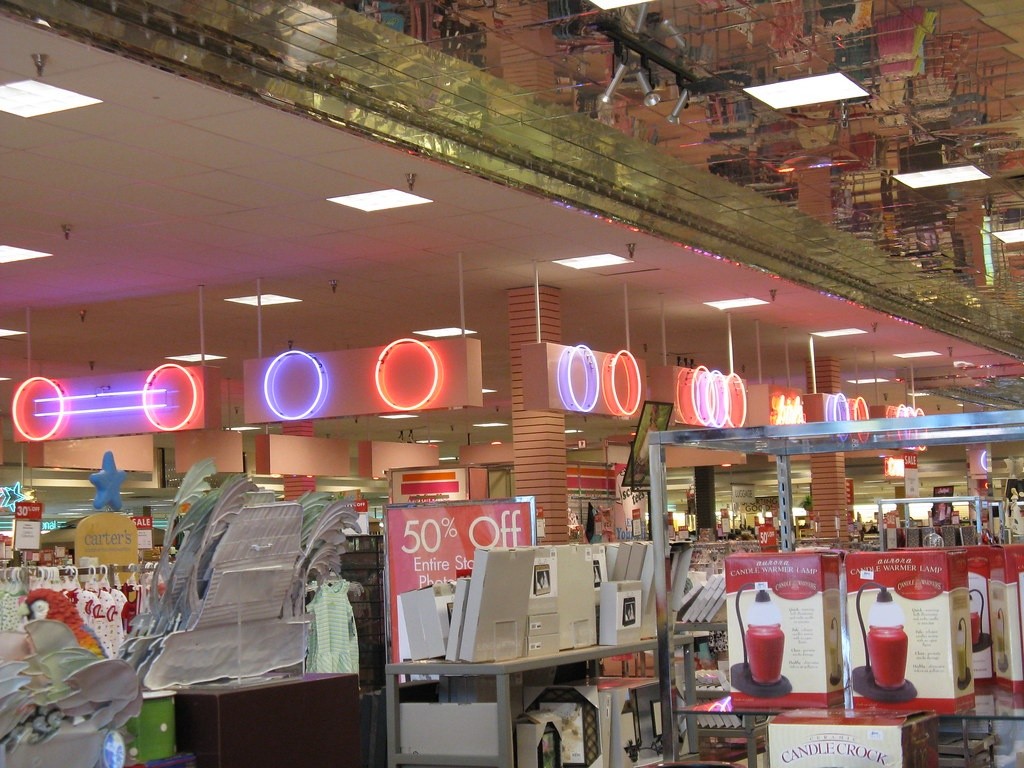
[397,541,1024,767]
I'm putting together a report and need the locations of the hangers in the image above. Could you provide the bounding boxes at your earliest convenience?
[317,566,343,590]
[0,562,164,595]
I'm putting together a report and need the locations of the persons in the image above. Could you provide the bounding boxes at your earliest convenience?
[634,405,659,480]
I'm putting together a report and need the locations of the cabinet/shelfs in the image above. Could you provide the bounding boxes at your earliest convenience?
[647,409,1024,768]
[385,635,699,768]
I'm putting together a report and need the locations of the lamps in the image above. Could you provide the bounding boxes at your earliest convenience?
[0,244,54,264]
[325,188,434,213]
[0,79,103,119]
[551,253,634,270]
[0,294,942,453]
